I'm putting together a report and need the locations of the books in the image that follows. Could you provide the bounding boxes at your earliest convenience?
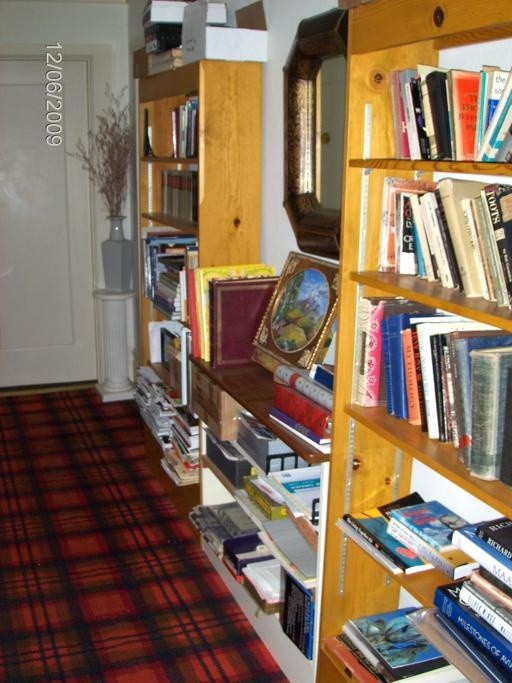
[376,64,512,307]
[355,295,512,486]
[132,96,201,488]
[328,491,511,683]
[187,262,335,659]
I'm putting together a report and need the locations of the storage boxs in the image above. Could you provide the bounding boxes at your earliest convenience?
[132,0,271,80]
[234,410,310,473]
[205,428,251,489]
[188,362,244,442]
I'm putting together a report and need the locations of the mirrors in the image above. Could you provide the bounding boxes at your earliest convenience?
[284,7,349,258]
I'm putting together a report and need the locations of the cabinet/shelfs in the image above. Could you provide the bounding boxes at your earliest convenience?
[135,58,262,365]
[187,353,331,683]
[313,2,512,682]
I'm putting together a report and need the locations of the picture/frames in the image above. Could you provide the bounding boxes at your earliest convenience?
[251,252,341,372]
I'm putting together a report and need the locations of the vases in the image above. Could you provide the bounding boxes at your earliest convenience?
[100,215,135,293]
